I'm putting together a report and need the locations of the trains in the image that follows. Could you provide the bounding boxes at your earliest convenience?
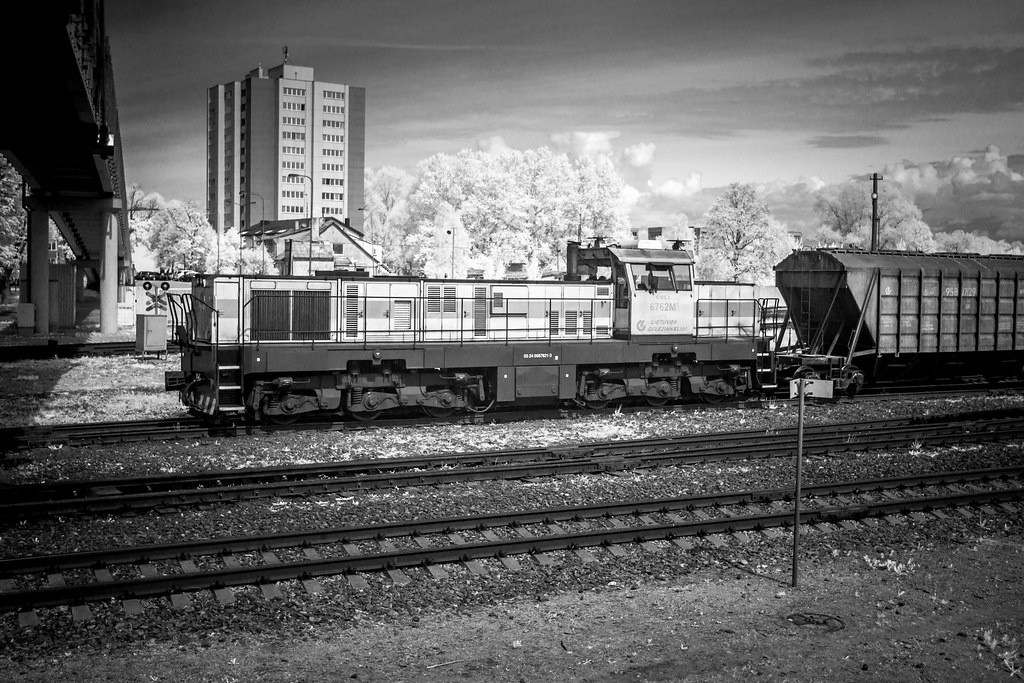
[163,239,1024,425]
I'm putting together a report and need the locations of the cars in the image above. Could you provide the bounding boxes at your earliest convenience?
[134,268,201,281]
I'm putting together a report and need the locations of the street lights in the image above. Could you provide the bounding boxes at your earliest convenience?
[447,226,455,278]
[239,192,265,275]
[358,207,375,275]
[556,242,562,281]
[224,198,242,274]
[288,173,314,276]
